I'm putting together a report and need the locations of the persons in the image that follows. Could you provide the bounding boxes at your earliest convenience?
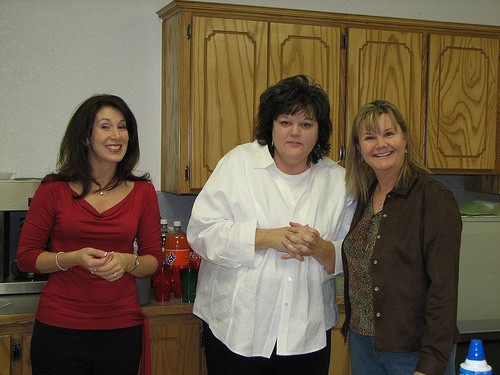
[341,100,461,375]
[17,94,163,375]
[186,74,358,375]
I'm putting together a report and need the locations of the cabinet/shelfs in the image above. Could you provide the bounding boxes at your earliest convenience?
[1,298,354,375]
[156,0,345,197]
[343,13,499,194]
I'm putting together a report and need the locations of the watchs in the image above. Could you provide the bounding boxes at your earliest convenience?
[128,254,141,273]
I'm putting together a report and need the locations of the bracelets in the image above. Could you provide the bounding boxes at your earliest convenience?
[56,252,67,271]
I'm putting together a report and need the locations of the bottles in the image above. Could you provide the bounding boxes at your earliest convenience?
[459,339,493,375]
[151,220,201,304]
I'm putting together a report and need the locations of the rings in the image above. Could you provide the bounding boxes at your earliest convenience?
[113,273,116,278]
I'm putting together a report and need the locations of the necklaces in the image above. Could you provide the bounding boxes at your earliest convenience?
[90,181,117,196]
[378,185,384,208]
